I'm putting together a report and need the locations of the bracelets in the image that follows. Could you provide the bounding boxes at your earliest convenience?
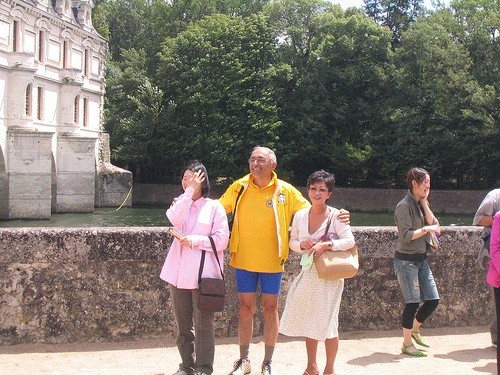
[328,241,332,251]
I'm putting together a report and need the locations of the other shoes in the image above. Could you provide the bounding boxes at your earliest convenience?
[261,361,271,375]
[173,368,210,375]
[229,358,251,375]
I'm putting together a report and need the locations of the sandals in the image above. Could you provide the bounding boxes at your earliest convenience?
[412,330,431,347]
[301,369,337,375]
[401,343,428,357]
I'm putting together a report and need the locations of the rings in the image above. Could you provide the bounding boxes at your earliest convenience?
[314,253,316,257]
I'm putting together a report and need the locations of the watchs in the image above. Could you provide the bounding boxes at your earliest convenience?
[422,226,427,235]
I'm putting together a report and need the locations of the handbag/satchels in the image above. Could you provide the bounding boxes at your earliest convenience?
[197,235,227,313]
[426,230,441,256]
[315,209,359,278]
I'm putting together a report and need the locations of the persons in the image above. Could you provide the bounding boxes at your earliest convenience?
[472,188,500,348]
[169,146,350,375]
[486,210,500,375]
[278,170,355,375]
[159,160,230,375]
[393,167,441,357]
